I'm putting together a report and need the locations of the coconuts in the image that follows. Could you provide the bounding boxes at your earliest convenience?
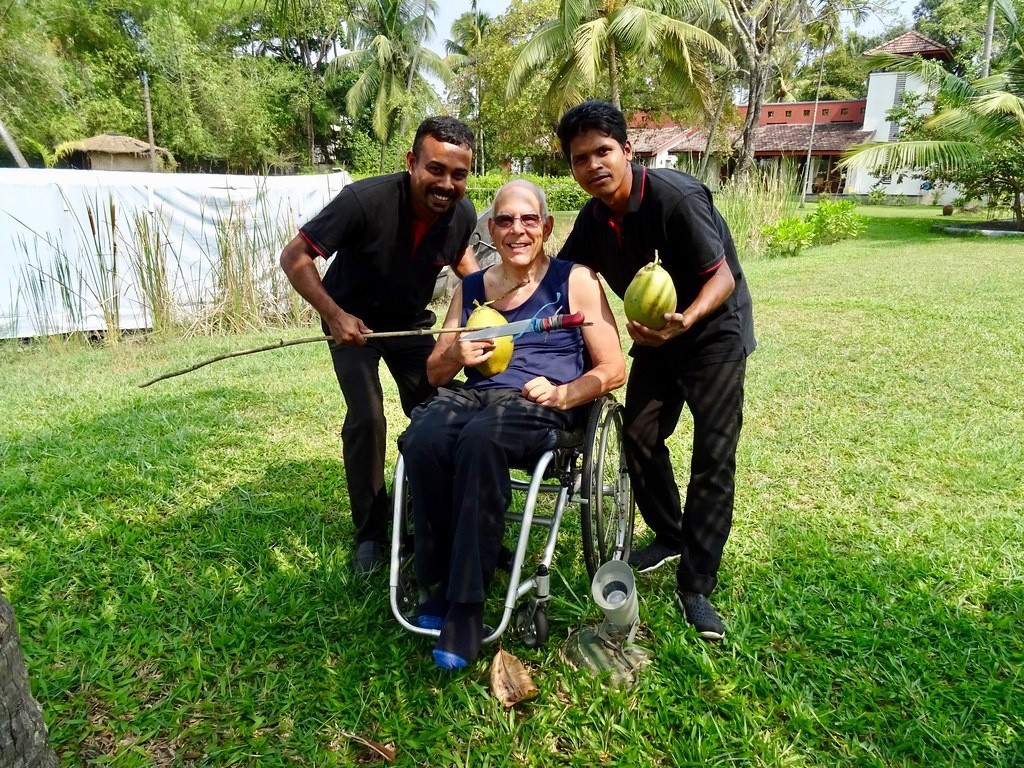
[465,304,514,376]
[624,263,677,331]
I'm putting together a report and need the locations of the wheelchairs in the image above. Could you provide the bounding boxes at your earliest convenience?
[389,391,636,654]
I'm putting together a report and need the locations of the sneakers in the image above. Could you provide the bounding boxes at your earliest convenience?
[673,585,726,639]
[629,535,682,572]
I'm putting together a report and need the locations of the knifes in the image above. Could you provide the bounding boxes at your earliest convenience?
[458,311,585,341]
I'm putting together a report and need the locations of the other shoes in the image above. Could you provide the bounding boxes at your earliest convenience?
[355,535,389,575]
[412,595,444,630]
[432,633,482,669]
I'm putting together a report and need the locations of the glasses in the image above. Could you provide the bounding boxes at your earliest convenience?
[492,214,544,228]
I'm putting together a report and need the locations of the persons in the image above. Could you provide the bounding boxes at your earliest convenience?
[280,115,480,576]
[400,179,626,669]
[557,101,755,639]
